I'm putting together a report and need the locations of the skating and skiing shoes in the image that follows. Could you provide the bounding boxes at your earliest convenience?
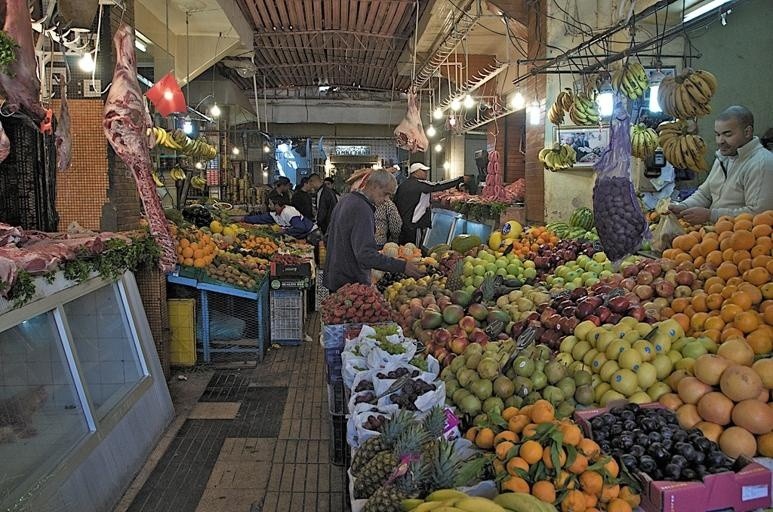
[221,250,270,270]
[347,322,429,372]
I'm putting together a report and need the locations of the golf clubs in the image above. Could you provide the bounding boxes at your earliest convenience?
[546,207,604,242]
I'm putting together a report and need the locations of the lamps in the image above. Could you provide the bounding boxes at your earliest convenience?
[210,31,223,118]
[647,9,668,113]
[424,38,474,152]
[511,63,526,110]
[77,49,94,73]
[596,39,615,117]
[531,76,541,126]
[231,110,240,154]
[162,1,175,100]
[182,9,193,135]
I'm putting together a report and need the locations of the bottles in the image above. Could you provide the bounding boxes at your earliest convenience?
[410,162,430,173]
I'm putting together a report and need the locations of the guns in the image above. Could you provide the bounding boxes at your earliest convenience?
[538,62,719,172]
[400,487,556,511]
[145,128,218,190]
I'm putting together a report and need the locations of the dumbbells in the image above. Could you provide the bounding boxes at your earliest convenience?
[382,237,417,259]
[426,234,483,258]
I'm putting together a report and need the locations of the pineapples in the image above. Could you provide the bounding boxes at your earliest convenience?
[399,295,488,366]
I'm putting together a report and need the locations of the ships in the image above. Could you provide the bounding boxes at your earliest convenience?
[483,150,506,204]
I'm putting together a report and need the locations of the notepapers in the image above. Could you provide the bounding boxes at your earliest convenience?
[445,264,464,290]
[347,406,443,512]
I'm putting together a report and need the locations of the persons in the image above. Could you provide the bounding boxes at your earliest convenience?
[661,105,773,225]
[229,174,342,264]
[321,169,427,295]
[345,162,471,250]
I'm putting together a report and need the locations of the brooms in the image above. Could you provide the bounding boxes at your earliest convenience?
[385,273,446,291]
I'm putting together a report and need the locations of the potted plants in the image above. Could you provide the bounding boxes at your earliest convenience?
[553,126,611,171]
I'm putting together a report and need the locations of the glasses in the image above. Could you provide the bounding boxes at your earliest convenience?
[531,76,541,126]
[596,39,615,117]
[182,9,193,135]
[424,38,474,152]
[210,31,223,118]
[77,49,94,73]
[231,110,240,154]
[647,9,668,113]
[162,1,175,100]
[511,63,526,110]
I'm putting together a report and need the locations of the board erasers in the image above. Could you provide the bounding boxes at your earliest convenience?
[395,286,451,301]
[205,264,257,292]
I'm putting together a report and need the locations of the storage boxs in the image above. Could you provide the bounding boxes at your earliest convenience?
[575,407,773,511]
[151,232,273,300]
[428,190,526,245]
[318,313,394,470]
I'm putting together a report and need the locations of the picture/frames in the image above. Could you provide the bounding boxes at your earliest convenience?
[553,126,611,171]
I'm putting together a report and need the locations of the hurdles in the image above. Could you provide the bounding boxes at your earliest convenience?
[442,338,594,418]
